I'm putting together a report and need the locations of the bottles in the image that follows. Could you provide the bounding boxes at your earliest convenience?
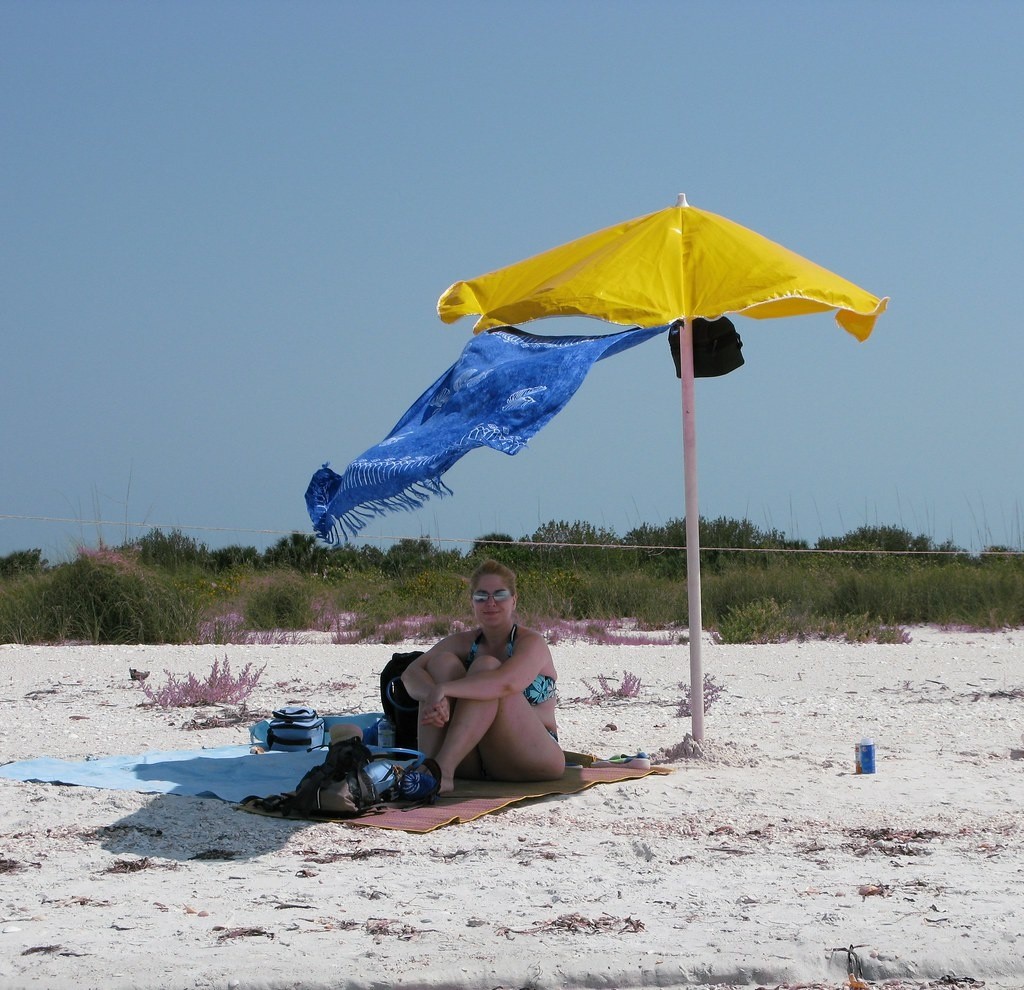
[378,715,397,748]
[860,738,876,773]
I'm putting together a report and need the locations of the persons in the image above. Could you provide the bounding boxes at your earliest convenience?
[399,560,565,793]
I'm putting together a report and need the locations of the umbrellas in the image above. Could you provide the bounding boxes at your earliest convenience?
[436,193,891,739]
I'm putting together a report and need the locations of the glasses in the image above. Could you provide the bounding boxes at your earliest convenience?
[472,590,513,602]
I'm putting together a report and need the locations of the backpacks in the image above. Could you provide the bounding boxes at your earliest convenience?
[267,707,325,752]
[281,736,388,819]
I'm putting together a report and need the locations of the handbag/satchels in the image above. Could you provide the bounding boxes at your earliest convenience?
[380,651,427,746]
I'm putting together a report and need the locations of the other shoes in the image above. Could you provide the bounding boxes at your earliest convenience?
[566,761,583,769]
[590,753,651,770]
